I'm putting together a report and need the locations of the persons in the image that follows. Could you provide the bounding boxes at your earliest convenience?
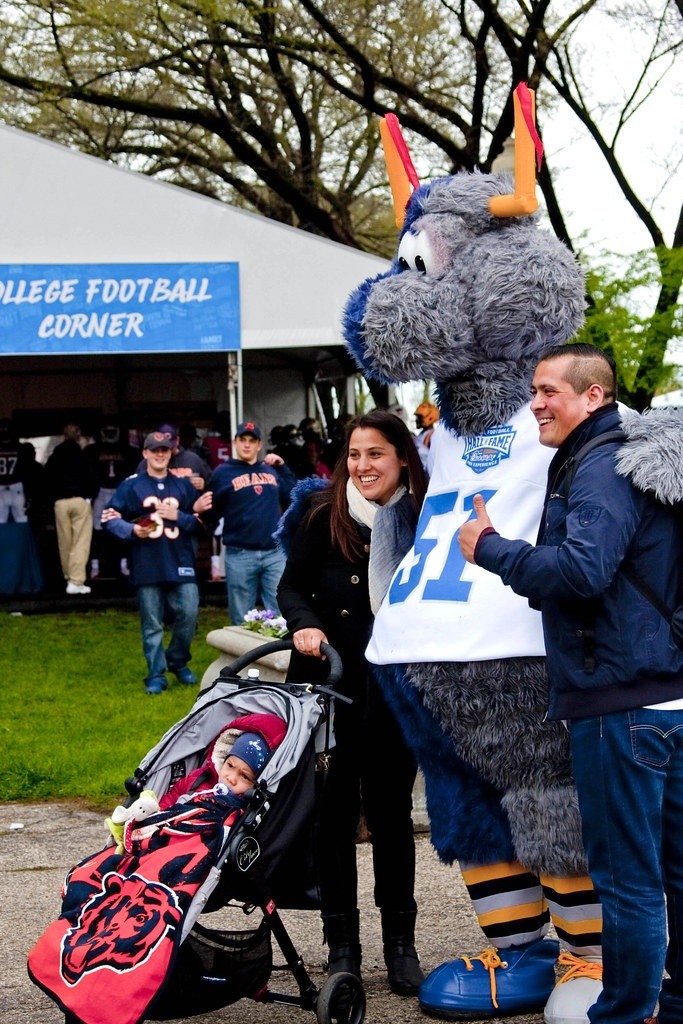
[257,412,354,482]
[456,342,682,1024]
[190,420,297,622]
[100,433,209,694]
[0,416,233,599]
[161,713,287,824]
[275,408,426,995]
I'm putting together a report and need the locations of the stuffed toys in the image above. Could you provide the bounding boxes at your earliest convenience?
[336,81,683,1023]
[105,789,162,855]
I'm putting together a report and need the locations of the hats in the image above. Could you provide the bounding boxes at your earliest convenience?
[144,435,172,451]
[160,426,178,448]
[236,423,263,442]
[223,733,270,777]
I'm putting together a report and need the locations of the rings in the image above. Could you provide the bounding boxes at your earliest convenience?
[299,640,304,643]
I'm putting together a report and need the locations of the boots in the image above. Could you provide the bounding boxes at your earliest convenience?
[320,907,363,982]
[380,907,425,995]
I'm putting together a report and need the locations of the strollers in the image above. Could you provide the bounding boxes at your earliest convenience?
[67,636,367,1024]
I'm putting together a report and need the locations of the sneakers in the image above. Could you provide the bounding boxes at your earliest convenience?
[65,580,92,595]
[144,678,172,693]
[166,663,198,684]
